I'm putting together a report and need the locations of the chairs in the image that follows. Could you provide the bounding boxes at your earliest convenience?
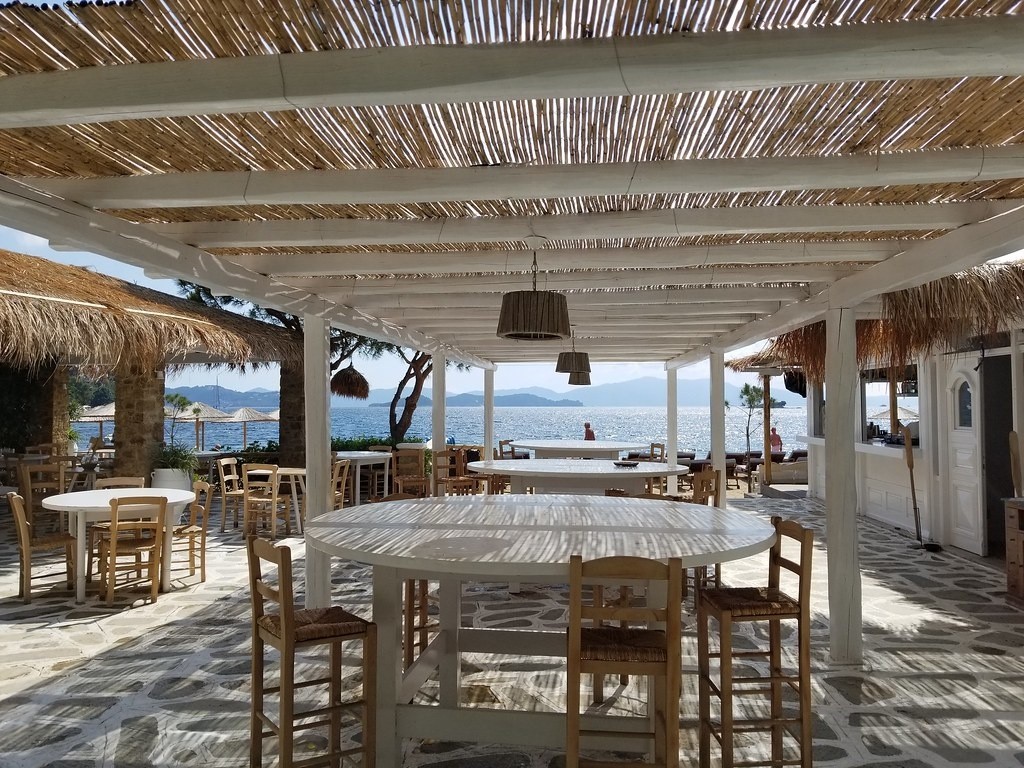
[0,438,516,768]
[567,443,817,768]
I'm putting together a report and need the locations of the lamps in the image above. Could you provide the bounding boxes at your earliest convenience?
[568,373,591,385]
[554,324,592,373]
[495,235,572,343]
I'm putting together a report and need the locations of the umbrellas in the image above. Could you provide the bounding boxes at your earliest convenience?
[166,402,280,450]
[70,402,173,439]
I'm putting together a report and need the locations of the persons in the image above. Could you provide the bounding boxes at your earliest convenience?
[770,427,783,452]
[212,445,221,452]
[579,423,596,460]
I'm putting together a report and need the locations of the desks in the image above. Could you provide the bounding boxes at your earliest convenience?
[0,454,50,492]
[509,440,651,460]
[467,461,690,497]
[304,494,777,768]
[41,487,196,602]
[74,449,116,458]
[63,466,112,493]
[247,468,306,534]
[335,450,393,506]
[196,451,221,483]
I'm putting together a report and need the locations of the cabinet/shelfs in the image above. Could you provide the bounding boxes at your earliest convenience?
[1000,496,1024,605]
